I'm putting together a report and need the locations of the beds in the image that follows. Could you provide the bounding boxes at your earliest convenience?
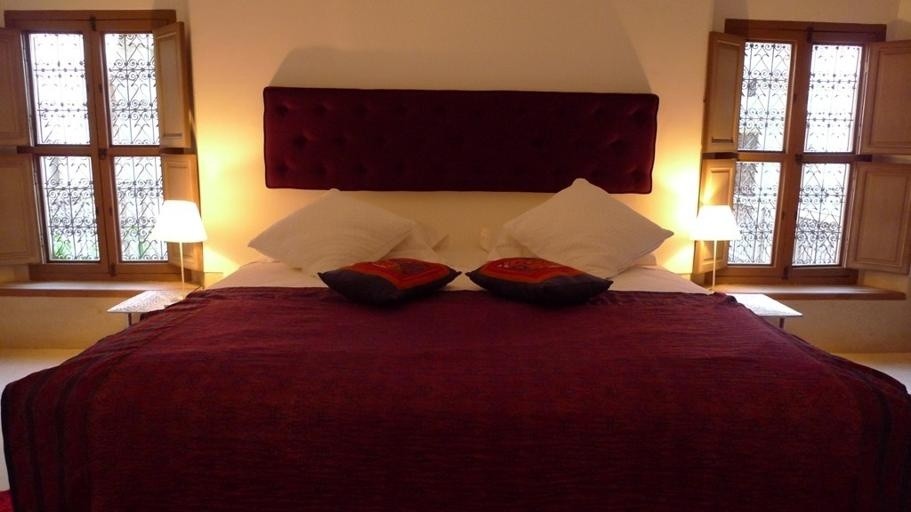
[0,85,911,512]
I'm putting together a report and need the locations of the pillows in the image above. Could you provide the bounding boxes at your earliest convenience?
[465,257,613,308]
[318,258,462,304]
[509,179,674,284]
[251,188,413,274]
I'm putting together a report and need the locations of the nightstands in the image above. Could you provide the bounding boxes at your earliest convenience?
[105,290,200,327]
[729,294,803,328]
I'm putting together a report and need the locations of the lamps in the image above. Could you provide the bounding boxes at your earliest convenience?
[147,200,209,306]
[688,205,742,292]
